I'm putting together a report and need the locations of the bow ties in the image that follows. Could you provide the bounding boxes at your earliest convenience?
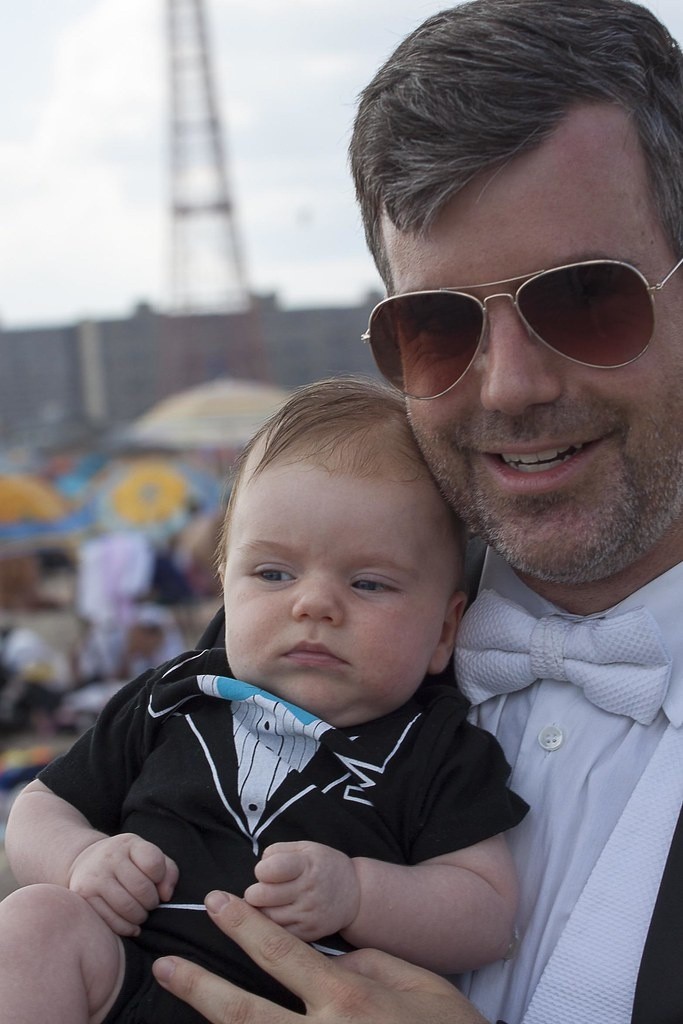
[451,588,672,727]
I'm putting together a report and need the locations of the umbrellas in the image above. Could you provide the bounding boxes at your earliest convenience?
[113,377,300,445]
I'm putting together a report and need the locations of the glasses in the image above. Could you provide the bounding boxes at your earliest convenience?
[360,259,682,401]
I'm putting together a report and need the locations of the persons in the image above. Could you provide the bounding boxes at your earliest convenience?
[0,0,683,1024]
